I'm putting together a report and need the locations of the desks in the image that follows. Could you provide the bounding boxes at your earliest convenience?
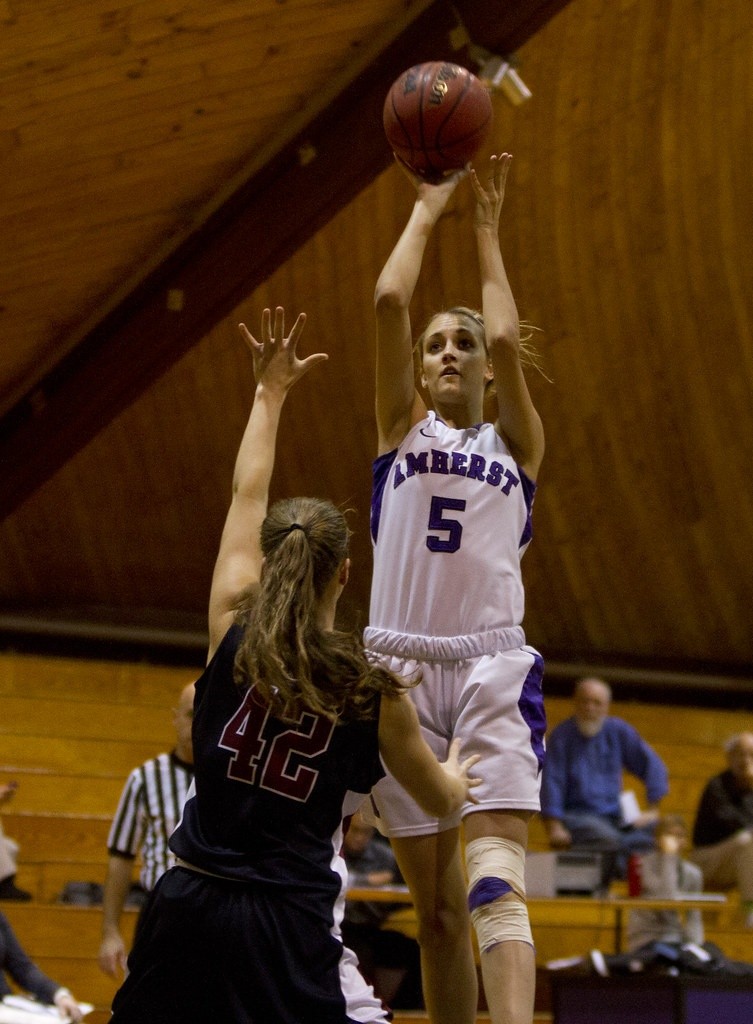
[344,884,727,955]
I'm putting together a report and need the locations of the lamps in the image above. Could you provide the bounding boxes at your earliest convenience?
[478,53,532,108]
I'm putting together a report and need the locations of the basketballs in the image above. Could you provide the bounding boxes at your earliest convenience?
[382,62,496,169]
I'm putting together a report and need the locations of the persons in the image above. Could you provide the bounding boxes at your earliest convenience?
[0,308,484,1024]
[540,678,753,907]
[363,152,545,1024]
